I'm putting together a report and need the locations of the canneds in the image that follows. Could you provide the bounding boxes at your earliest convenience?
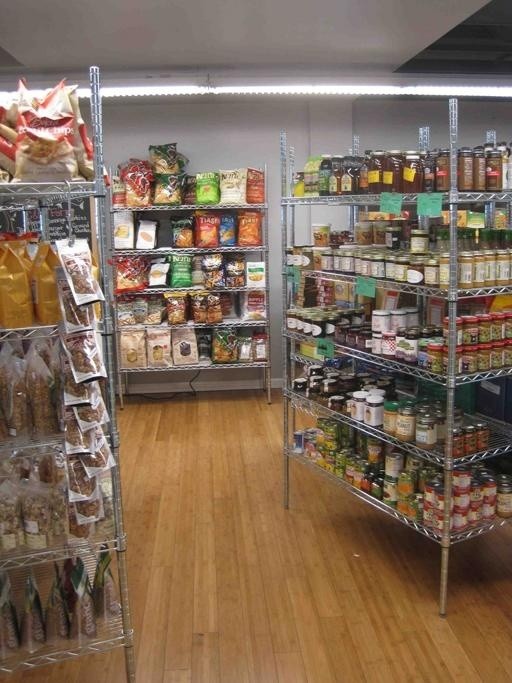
[284,244,313,269]
[367,149,509,193]
[320,226,512,289]
[286,306,512,533]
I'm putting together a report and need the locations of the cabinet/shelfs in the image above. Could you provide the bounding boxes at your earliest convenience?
[3,66,141,677]
[274,95,512,619]
[85,127,274,415]
